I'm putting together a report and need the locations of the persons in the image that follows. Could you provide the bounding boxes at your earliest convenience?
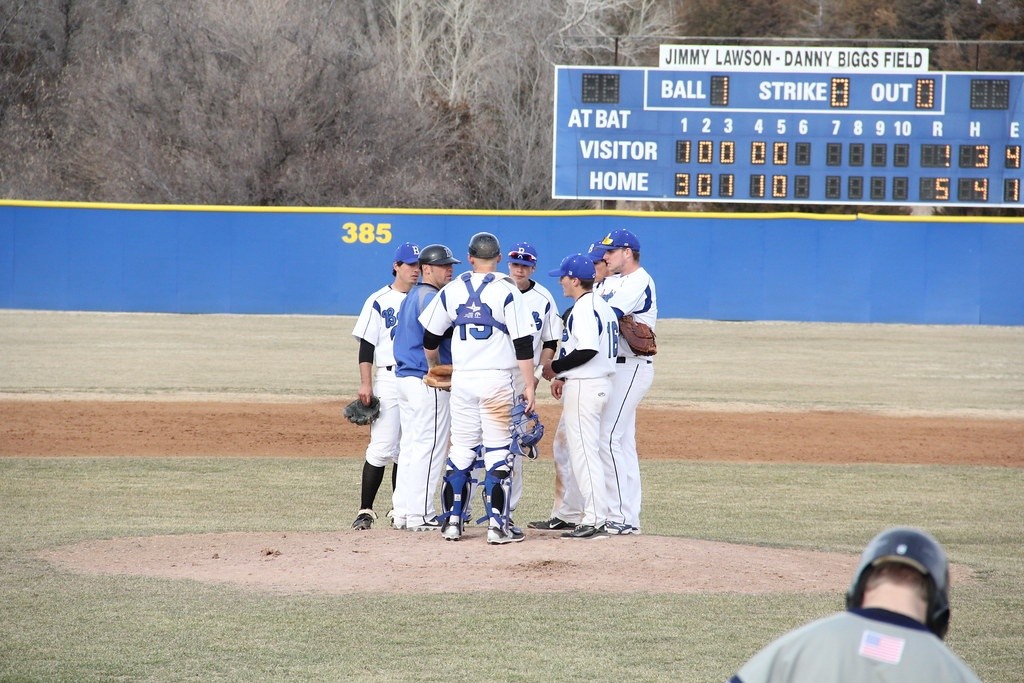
[351,227,660,543]
[729,526,981,683]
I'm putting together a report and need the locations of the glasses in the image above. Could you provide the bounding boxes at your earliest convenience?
[509,251,535,262]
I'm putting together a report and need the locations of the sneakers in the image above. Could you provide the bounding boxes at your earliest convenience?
[560,525,612,540]
[527,518,577,530]
[596,521,641,535]
[389,516,407,530]
[486,526,526,543]
[406,514,444,531]
[349,508,378,531]
[440,521,462,541]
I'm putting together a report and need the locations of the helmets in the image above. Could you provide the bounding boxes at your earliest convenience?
[596,229,641,251]
[467,231,501,259]
[843,527,951,637]
[417,244,462,269]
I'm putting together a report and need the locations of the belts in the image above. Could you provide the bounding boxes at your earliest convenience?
[615,356,652,365]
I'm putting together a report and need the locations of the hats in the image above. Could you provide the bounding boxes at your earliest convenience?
[392,241,422,264]
[585,239,610,261]
[547,253,597,279]
[506,241,538,267]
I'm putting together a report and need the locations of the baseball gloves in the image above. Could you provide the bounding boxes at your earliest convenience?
[620,315,658,356]
[343,397,381,427]
[423,365,453,389]
